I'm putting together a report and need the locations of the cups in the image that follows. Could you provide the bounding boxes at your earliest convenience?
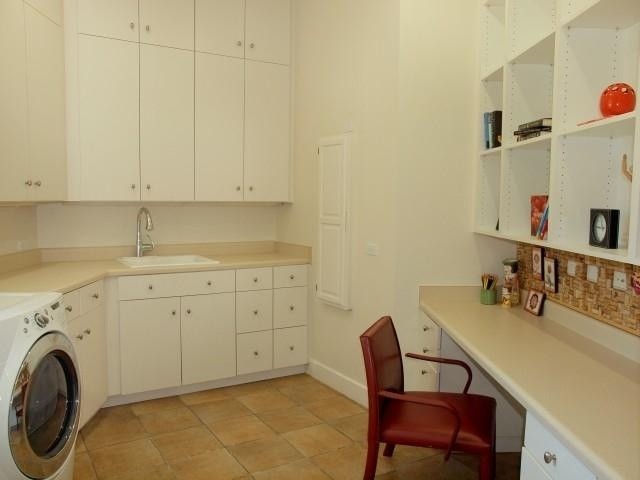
[480,288,497,305]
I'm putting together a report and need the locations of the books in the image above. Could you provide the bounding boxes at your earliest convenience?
[513,128,553,136]
[516,130,552,142]
[517,117,552,132]
[483,111,503,148]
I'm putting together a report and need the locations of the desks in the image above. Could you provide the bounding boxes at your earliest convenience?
[412,308,639,479]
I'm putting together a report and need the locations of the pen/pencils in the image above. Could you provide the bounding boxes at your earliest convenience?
[481,272,498,290]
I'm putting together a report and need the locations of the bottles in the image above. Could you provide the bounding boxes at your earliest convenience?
[501,260,520,308]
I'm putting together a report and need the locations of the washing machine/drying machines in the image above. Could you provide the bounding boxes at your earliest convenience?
[0,292,81,480]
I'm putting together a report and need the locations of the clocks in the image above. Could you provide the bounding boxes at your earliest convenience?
[588,208,621,250]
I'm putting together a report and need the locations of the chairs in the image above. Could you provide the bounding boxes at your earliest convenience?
[356,311,500,479]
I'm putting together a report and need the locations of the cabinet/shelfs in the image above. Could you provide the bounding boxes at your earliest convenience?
[474,0,640,268]
[233,261,315,386]
[110,267,237,406]
[61,273,108,438]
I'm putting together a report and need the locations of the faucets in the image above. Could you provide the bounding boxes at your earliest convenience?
[136,207,155,257]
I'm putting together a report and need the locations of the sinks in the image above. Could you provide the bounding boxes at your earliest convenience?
[115,255,219,267]
